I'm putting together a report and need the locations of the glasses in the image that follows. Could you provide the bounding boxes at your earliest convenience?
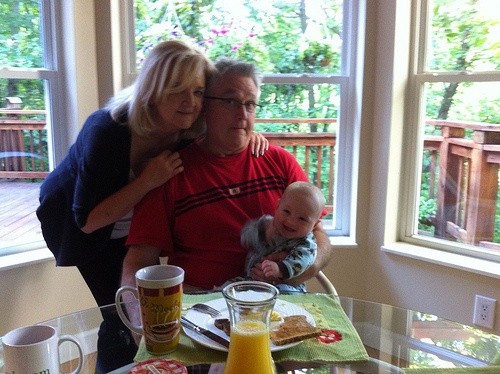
[207,93,262,115]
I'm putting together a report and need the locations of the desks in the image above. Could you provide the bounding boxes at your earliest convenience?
[0,291,500,374]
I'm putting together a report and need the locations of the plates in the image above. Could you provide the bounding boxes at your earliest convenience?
[181,297,315,352]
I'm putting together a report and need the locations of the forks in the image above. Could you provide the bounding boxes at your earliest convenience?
[180,304,220,318]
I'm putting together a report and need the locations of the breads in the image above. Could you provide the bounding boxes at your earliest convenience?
[271,315,322,345]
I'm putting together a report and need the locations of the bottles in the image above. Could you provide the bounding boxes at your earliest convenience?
[222,281,279,374]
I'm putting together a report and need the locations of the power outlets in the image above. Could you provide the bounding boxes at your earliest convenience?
[473,294,497,330]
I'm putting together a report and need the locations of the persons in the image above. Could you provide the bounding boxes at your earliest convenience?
[214,181,324,294]
[36,41,268,374]
[122,56,332,348]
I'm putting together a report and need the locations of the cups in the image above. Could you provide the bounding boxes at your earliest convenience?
[115,265,185,356]
[0,322,85,374]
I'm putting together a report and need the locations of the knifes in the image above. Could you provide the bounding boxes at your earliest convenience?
[180,316,229,350]
